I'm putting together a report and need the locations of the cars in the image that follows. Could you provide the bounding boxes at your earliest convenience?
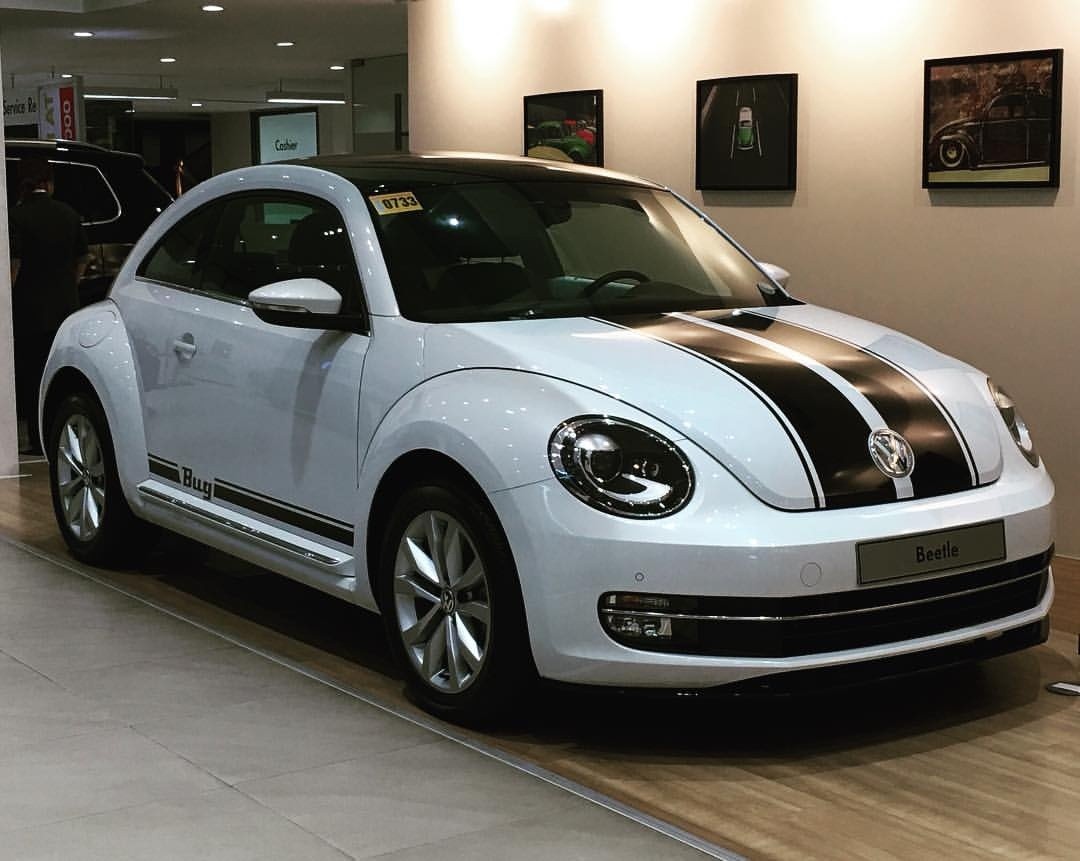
[526,118,597,163]
[928,79,1051,170]
[38,151,1059,731]
[737,106,756,150]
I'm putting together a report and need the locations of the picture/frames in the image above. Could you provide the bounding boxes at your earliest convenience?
[523,89,603,168]
[696,73,798,190]
[922,48,1064,189]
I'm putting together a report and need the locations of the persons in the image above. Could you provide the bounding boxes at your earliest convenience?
[9,162,85,455]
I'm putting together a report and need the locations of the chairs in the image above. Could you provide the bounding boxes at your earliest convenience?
[200,202,546,309]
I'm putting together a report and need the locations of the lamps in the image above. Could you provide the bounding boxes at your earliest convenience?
[266,79,346,105]
[83,75,178,101]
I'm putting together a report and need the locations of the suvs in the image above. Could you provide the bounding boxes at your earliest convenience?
[4,138,176,298]
